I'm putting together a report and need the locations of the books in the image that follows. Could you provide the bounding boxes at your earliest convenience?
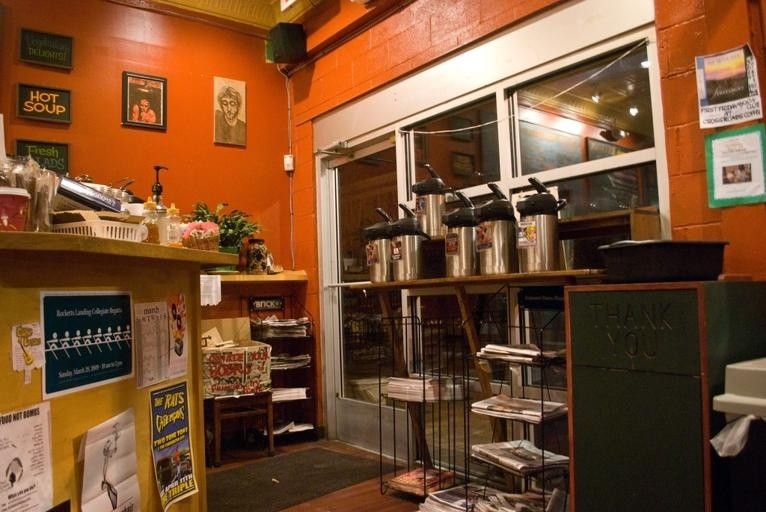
[269,353,312,370]
[471,393,568,425]
[263,419,314,436]
[474,488,564,512]
[476,343,558,364]
[418,481,498,512]
[389,466,452,497]
[272,386,307,401]
[387,376,464,402]
[252,316,310,338]
[471,438,569,476]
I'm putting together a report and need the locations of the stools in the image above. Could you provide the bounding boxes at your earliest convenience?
[204,389,275,467]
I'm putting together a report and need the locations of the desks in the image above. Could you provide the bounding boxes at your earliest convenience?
[328,269,606,492]
[0,227,239,512]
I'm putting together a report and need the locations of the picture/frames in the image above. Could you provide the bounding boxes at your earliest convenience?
[121,71,167,131]
[18,26,73,70]
[16,80,72,124]
[13,137,71,177]
[213,77,246,147]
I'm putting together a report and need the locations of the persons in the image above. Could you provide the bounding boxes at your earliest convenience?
[132,93,156,124]
[215,86,245,145]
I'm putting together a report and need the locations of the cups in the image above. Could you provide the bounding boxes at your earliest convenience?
[559,239,574,271]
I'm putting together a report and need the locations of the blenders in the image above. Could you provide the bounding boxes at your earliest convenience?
[412,165,454,237]
[441,191,479,276]
[474,182,517,274]
[390,204,431,281]
[516,177,568,272]
[364,208,393,282]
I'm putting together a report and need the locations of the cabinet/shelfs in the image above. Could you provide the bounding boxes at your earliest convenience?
[564,282,765,512]
[238,292,319,447]
